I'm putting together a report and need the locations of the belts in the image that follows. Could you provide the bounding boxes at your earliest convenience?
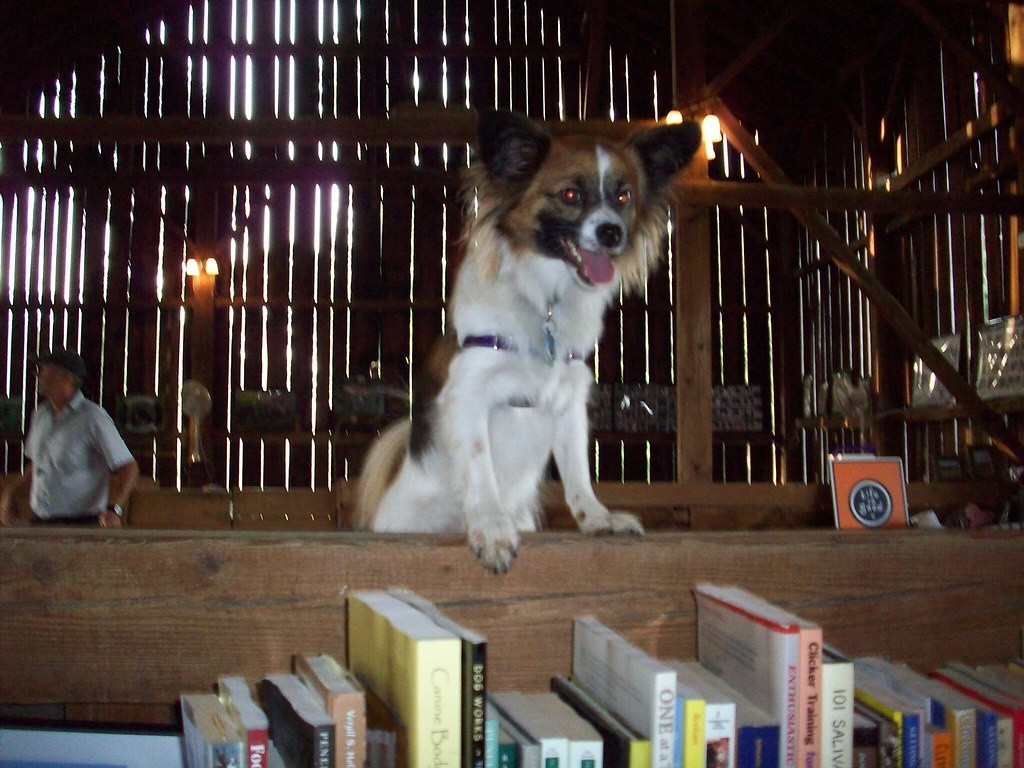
[30,513,100,525]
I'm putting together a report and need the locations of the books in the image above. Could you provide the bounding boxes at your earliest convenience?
[177,587,1024,768]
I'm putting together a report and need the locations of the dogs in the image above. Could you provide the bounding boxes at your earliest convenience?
[350,108,702,575]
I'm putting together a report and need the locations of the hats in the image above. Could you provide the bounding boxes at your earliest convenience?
[31,348,86,377]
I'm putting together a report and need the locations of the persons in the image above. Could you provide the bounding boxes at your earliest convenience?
[0,350,138,528]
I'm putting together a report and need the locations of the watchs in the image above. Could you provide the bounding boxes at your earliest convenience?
[107,503,124,518]
